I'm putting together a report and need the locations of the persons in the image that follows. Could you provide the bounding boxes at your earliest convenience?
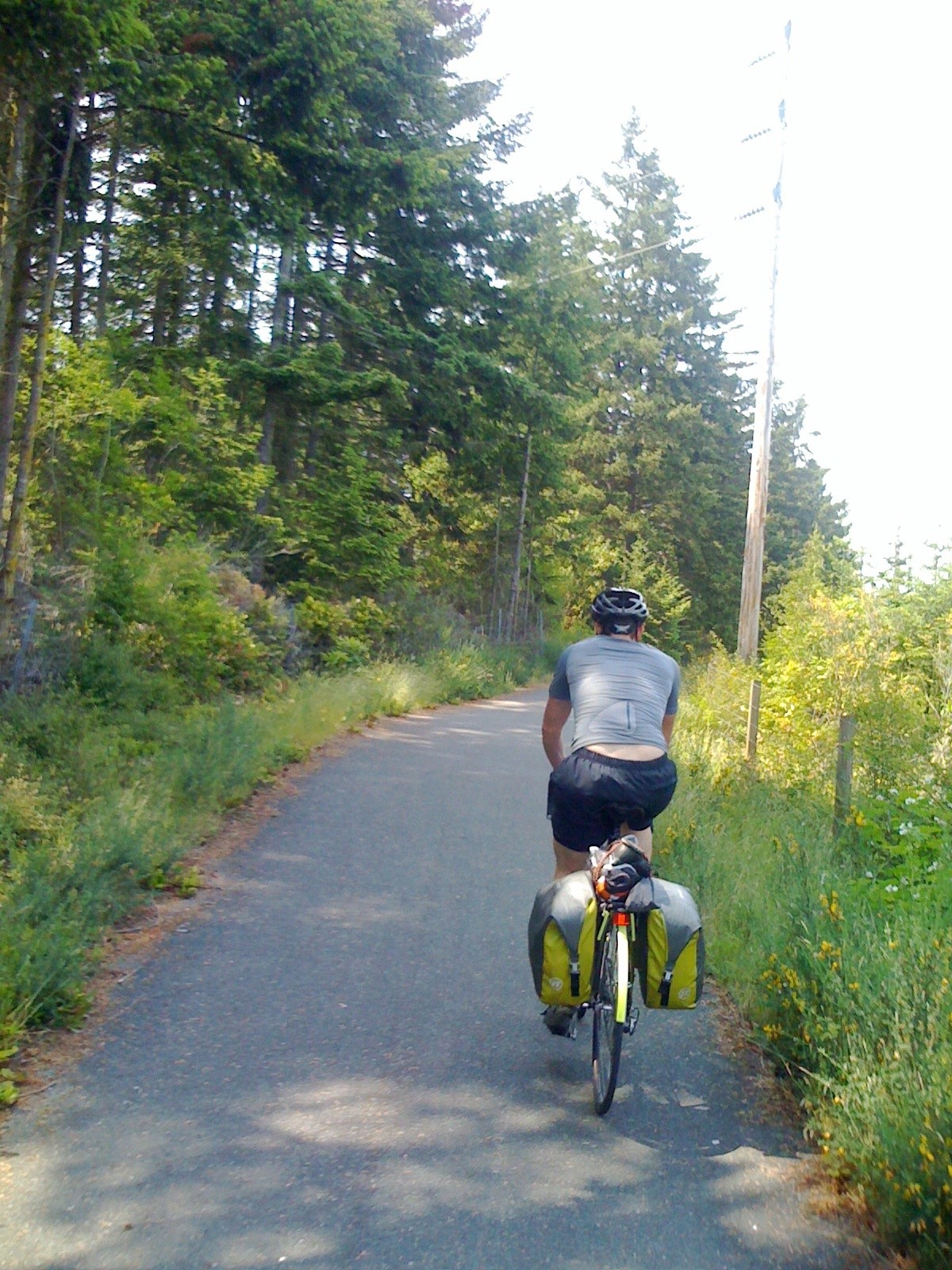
[542,586,681,1037]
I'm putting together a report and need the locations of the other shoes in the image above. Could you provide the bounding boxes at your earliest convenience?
[542,1001,576,1033]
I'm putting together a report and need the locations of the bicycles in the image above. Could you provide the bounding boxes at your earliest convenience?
[542,800,659,1119]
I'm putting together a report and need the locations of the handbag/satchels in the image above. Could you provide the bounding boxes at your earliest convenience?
[529,868,706,1009]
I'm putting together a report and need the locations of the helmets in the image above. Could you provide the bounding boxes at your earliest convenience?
[590,586,649,623]
